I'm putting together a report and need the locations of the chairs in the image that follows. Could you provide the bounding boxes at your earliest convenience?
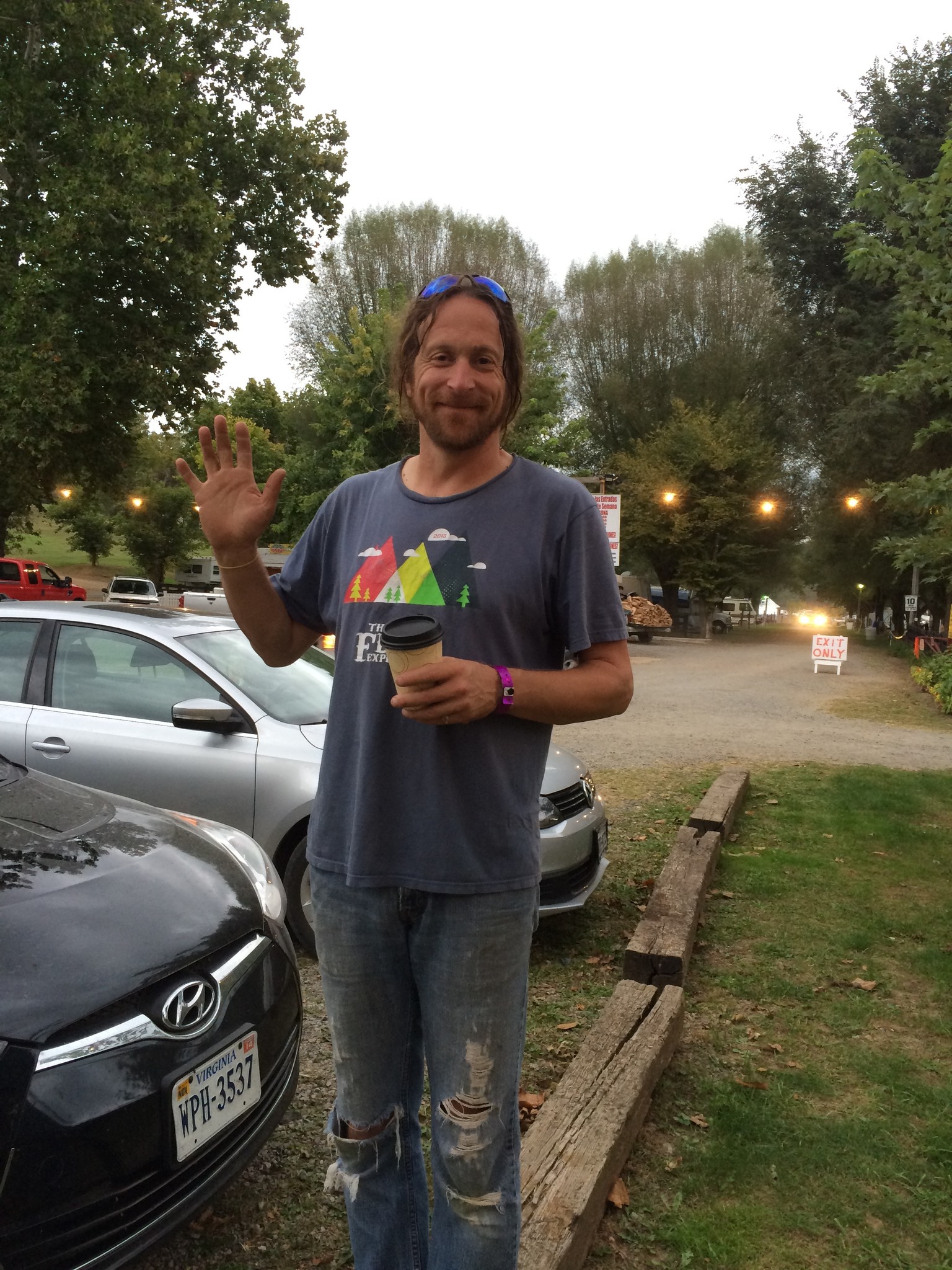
[59,643,171,717]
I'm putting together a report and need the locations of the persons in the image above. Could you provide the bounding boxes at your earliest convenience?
[177,271,635,1270]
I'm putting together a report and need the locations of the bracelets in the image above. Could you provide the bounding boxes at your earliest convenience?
[215,554,259,571]
[489,665,514,715]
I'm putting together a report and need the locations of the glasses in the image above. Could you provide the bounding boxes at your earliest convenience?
[416,273,514,306]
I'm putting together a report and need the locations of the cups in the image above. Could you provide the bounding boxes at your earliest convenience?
[380,615,445,711]
